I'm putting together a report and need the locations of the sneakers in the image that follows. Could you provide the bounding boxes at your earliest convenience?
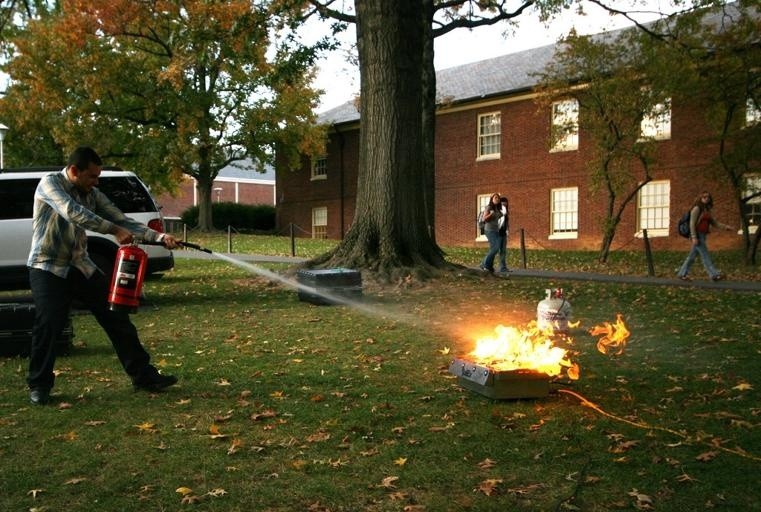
[479,264,513,272]
[679,273,691,281]
[712,274,725,280]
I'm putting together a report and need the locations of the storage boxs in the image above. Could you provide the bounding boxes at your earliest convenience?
[0,303,76,357]
[296,268,362,307]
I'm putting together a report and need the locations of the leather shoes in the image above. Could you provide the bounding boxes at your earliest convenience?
[30,386,47,404]
[133,373,177,392]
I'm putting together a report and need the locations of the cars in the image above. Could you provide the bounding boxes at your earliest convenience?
[0,168,173,307]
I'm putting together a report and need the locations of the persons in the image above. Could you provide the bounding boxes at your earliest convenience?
[478,192,514,273]
[675,190,736,282]
[25,146,185,407]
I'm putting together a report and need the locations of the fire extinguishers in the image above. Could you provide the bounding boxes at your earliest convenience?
[107,235,212,314]
[537,288,573,337]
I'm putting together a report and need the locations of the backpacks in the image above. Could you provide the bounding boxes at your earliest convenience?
[678,206,703,238]
[479,211,487,229]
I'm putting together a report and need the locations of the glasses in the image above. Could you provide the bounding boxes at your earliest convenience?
[703,196,709,198]
